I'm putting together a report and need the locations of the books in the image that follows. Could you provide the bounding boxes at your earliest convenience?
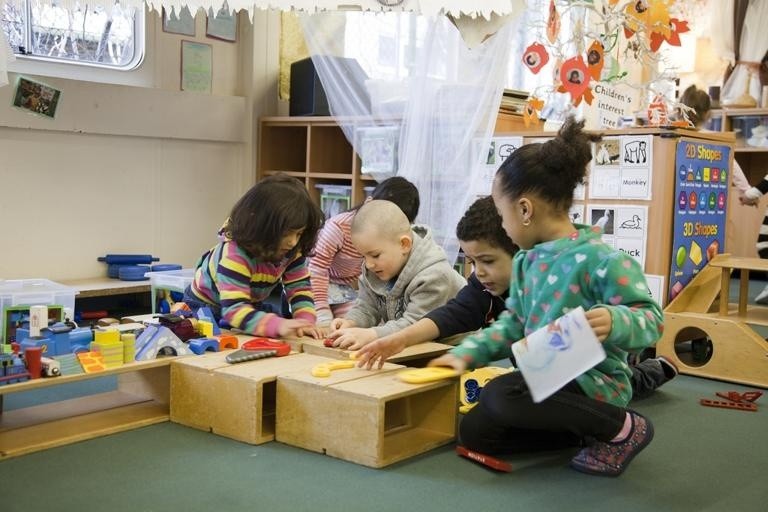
[455,445,564,472]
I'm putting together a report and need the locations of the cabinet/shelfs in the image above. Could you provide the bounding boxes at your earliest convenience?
[704,108,768,258]
[255,97,547,221]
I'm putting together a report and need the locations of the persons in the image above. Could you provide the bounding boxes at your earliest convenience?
[348,190,679,403]
[425,114,655,478]
[325,197,484,352]
[308,176,419,338]
[184,172,334,341]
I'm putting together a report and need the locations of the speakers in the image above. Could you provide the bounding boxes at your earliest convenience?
[289,55,371,116]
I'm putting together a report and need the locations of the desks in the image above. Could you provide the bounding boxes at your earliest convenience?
[467,128,736,312]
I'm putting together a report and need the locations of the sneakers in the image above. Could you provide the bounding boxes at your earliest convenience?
[658,355,678,381]
[570,410,654,476]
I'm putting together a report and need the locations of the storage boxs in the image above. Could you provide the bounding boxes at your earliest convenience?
[143,267,195,314]
[0,278,80,344]
[313,126,399,220]
